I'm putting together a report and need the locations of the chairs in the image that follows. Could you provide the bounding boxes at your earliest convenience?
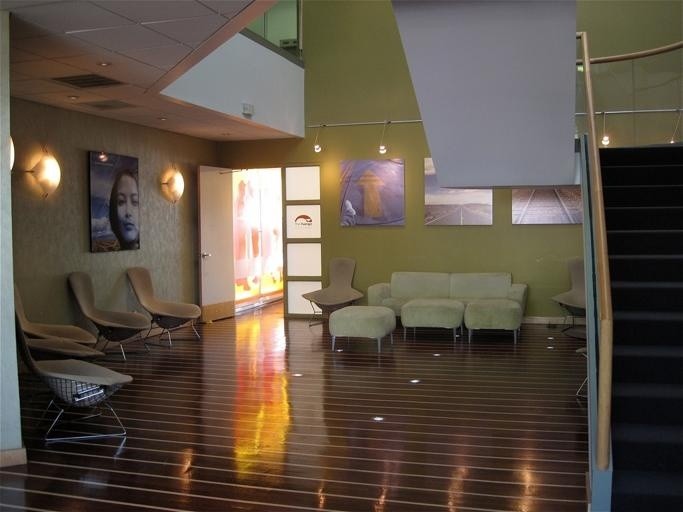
[126,267,202,347]
[301,256,363,325]
[66,269,152,362]
[26,337,104,360]
[17,329,133,441]
[12,282,97,346]
[551,257,587,343]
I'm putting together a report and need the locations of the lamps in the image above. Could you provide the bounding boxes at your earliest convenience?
[24,143,61,201]
[302,108,683,160]
[157,158,184,205]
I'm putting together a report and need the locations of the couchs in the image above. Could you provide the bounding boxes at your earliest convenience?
[462,300,522,347]
[328,304,395,355]
[366,270,528,319]
[400,297,463,346]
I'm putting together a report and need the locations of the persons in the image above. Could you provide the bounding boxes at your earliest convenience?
[107,166,139,251]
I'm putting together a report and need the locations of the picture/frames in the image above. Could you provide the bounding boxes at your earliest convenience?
[84,148,142,254]
